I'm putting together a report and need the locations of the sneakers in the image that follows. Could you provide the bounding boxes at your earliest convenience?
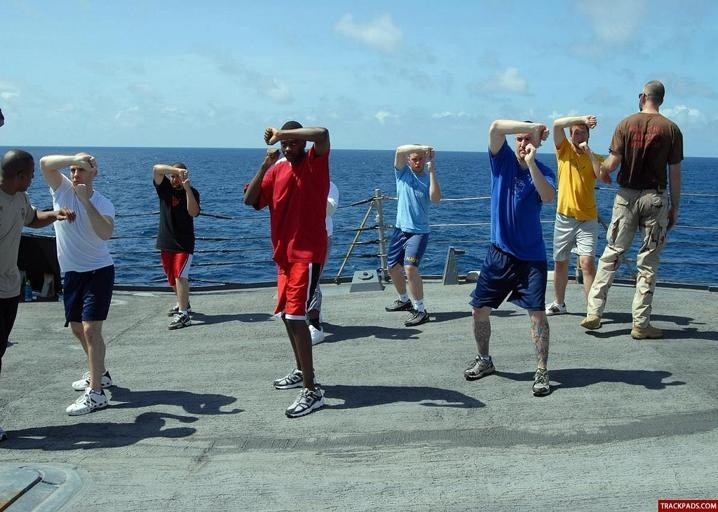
[274,367,315,390]
[631,325,663,339]
[532,368,548,396]
[285,388,323,417]
[67,389,107,415]
[386,300,410,311]
[582,315,599,329]
[403,310,428,326]
[168,314,191,328]
[544,302,566,315]
[167,302,192,315]
[307,324,325,346]
[464,356,494,380]
[71,370,111,391]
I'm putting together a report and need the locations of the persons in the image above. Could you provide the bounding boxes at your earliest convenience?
[546,115,611,314]
[385,145,440,328]
[0,109,77,376]
[305,181,340,345]
[465,119,557,395]
[40,152,114,416]
[152,162,201,331]
[243,121,330,419]
[582,80,684,338]
